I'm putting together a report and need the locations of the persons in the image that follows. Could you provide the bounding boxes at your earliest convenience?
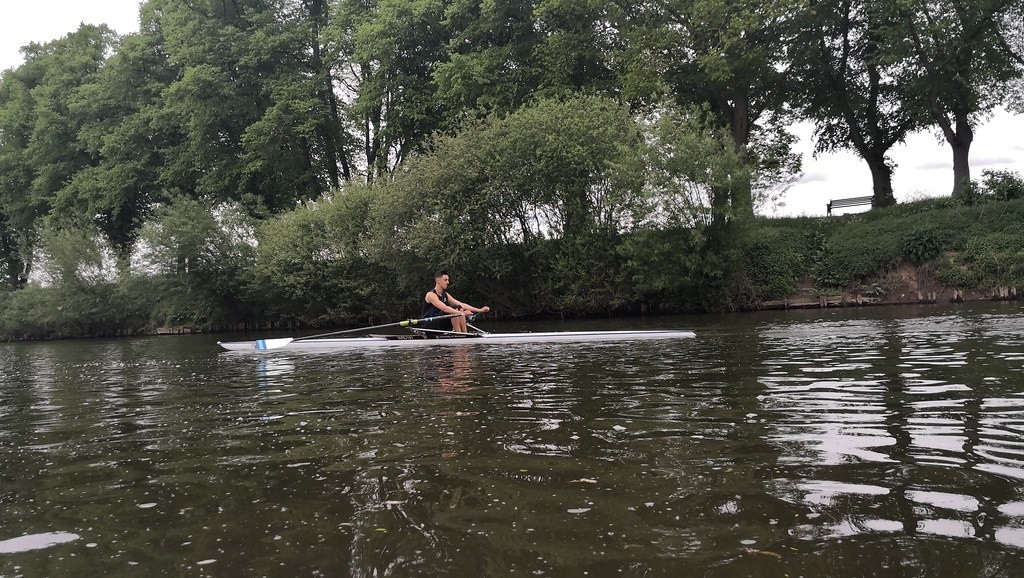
[422,271,490,333]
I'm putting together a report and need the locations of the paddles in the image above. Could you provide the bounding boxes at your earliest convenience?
[255,312,465,353]
[468,308,488,323]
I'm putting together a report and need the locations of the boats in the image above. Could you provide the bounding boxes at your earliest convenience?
[217,330,696,352]
